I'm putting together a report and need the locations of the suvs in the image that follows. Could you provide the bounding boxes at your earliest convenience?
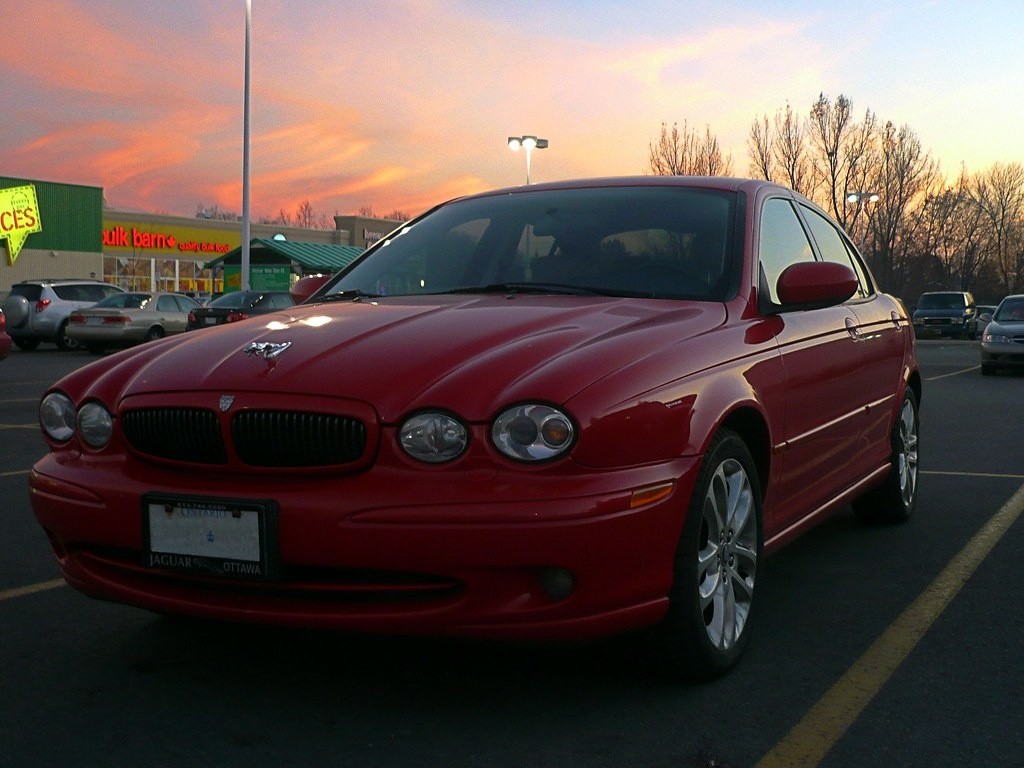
[1,277,131,353]
[909,291,979,340]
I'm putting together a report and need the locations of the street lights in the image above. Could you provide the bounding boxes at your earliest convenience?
[507,135,549,271]
[845,192,880,256]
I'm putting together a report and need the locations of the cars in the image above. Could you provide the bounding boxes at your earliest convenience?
[183,290,299,334]
[175,290,212,308]
[22,174,926,681]
[976,305,999,318]
[979,295,1024,374]
[63,290,205,353]
